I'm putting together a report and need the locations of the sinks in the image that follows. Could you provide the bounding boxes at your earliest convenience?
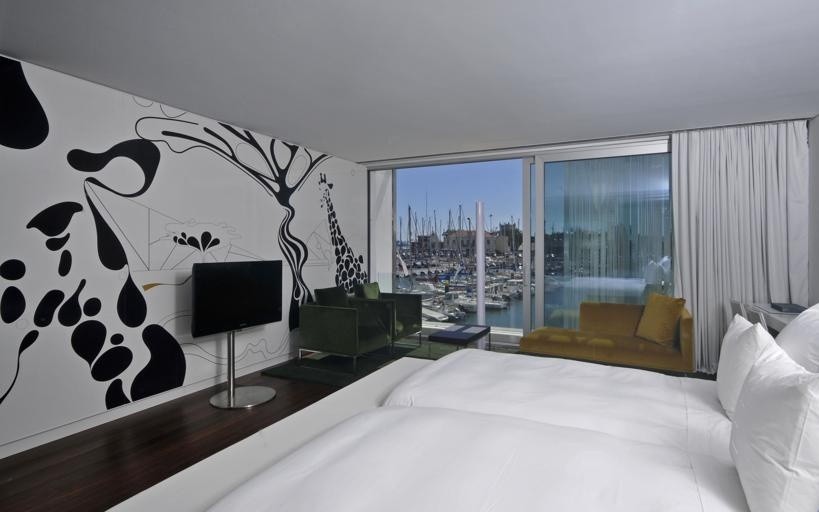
[348,284,423,348]
[300,282,395,371]
[515,293,696,376]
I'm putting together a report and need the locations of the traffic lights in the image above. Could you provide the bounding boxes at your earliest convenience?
[725,342,819,512]
[715,304,769,421]
[635,292,685,346]
[361,282,381,301]
[774,301,819,374]
[314,283,351,308]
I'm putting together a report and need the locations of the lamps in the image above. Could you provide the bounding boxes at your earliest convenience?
[745,307,770,334]
[730,299,748,320]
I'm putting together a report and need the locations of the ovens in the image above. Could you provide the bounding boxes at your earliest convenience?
[99,301,819,510]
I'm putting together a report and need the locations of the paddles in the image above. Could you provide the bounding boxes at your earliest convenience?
[261,341,417,386]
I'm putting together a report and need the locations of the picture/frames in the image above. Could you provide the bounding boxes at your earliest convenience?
[749,301,809,334]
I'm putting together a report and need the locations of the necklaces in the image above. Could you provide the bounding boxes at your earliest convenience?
[397,204,584,322]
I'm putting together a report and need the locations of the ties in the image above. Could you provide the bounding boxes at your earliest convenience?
[190,260,284,340]
